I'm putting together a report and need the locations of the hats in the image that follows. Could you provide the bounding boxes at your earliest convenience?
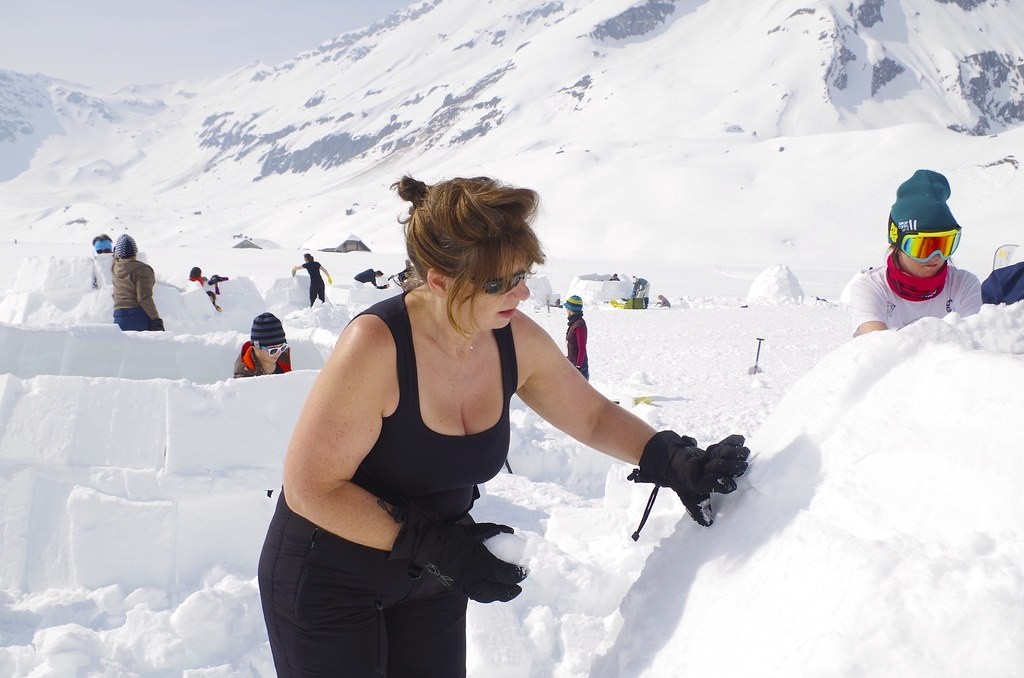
[632,276,638,284]
[115,234,138,258]
[563,295,583,312]
[250,312,288,346]
[888,169,962,247]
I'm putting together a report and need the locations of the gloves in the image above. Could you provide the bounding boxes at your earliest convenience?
[292,267,296,277]
[150,318,164,330]
[626,430,751,542]
[327,275,332,285]
[375,494,531,604]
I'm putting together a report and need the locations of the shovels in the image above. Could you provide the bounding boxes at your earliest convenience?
[749,338,764,373]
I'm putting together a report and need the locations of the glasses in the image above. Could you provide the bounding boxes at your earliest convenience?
[484,259,535,296]
[97,249,111,253]
[896,227,963,263]
[260,343,289,356]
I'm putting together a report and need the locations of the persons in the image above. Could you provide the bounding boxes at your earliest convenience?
[234,313,292,380]
[849,169,982,339]
[609,274,620,281]
[655,295,670,307]
[189,266,221,312]
[562,296,588,382]
[292,252,332,306]
[353,260,413,290]
[632,276,650,309]
[93,234,164,330]
[256,176,749,678]
